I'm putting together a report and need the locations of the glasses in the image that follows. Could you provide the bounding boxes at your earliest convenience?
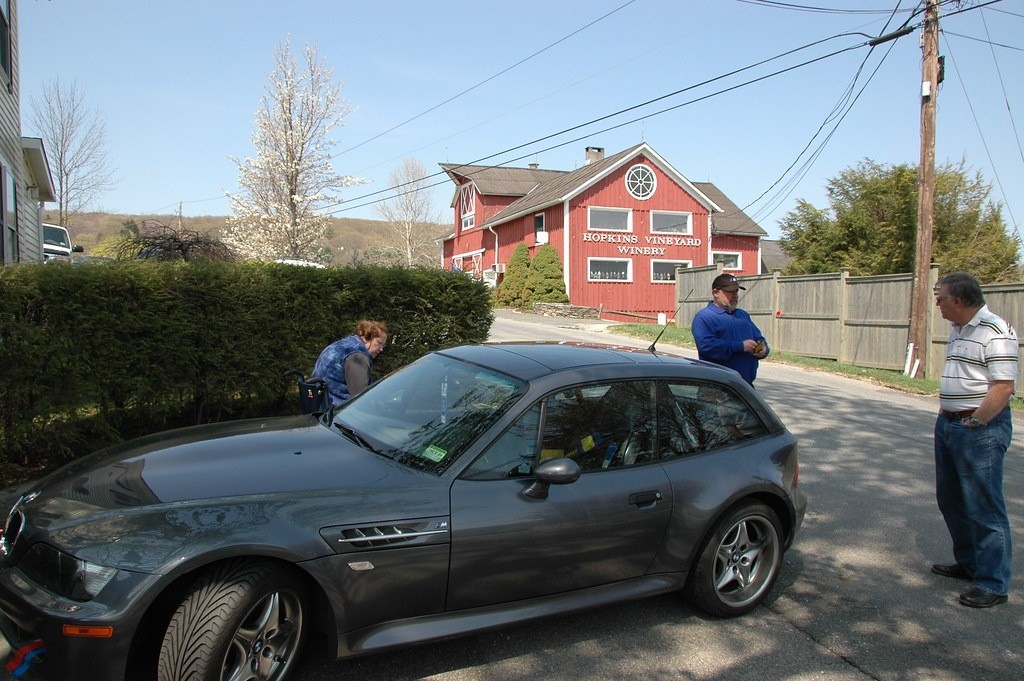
[372,335,386,349]
[936,296,955,303]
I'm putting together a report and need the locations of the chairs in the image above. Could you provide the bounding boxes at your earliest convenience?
[565,384,647,467]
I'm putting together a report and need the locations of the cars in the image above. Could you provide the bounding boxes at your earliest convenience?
[0,341,807,681]
[42,223,84,264]
[75,256,115,264]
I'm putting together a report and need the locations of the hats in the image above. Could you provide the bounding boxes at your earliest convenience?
[712,274,746,292]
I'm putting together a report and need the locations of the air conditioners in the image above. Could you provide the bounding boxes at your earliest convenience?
[492,263,506,273]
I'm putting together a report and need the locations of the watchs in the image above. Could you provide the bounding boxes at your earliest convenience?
[968,414,987,426]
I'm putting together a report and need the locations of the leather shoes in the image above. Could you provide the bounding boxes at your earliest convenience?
[930,564,974,582]
[959,588,1008,608]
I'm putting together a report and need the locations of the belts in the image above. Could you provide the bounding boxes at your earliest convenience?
[943,410,976,422]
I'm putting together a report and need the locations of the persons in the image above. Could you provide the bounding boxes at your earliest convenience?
[930,272,1019,607]
[691,273,770,401]
[311,320,387,407]
